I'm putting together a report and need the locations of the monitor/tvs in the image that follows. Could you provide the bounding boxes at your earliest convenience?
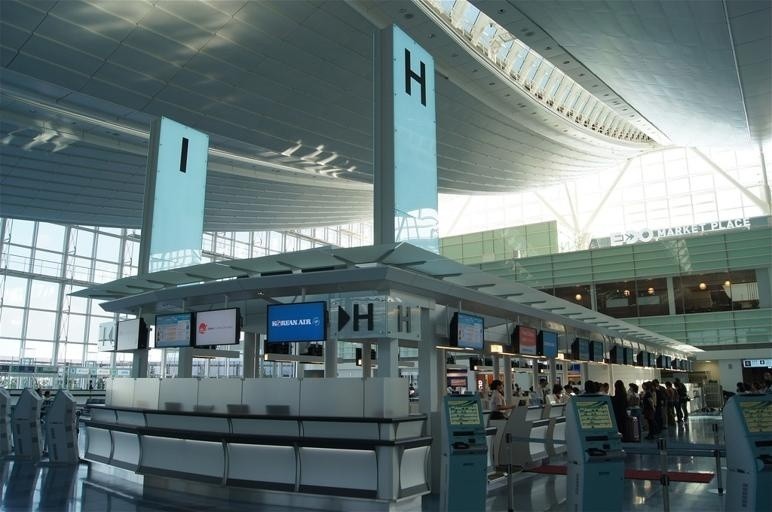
[267,301,326,344]
[578,337,689,370]
[117,317,141,351]
[576,401,614,429]
[518,325,537,356]
[447,399,481,425]
[155,312,193,348]
[456,312,484,350]
[739,400,772,433]
[542,330,558,357]
[195,307,238,346]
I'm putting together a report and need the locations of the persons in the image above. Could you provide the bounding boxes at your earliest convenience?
[44,391,50,398]
[489,379,516,420]
[409,384,415,402]
[737,372,772,393]
[36,388,42,398]
[514,377,688,442]
